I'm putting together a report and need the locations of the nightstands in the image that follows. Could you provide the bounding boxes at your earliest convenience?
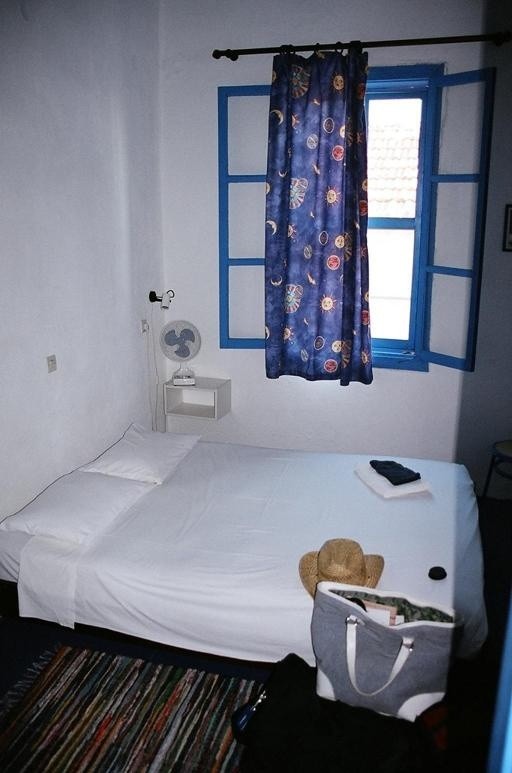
[164,375,232,421]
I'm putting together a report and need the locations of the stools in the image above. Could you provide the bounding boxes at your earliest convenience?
[480,440,512,510]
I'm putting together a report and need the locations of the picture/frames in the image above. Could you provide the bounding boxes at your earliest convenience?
[502,204,512,251]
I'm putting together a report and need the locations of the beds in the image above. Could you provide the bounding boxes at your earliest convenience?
[0,438,490,667]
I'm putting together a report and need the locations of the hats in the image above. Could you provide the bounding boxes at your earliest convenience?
[299,538,385,597]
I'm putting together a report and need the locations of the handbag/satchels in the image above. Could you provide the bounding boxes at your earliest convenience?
[312,582,464,723]
[232,654,436,771]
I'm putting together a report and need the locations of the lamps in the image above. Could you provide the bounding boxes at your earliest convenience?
[149,290,175,309]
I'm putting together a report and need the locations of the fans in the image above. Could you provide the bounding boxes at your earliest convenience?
[159,320,201,385]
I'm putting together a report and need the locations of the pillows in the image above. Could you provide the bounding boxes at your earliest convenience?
[0,469,157,544]
[78,420,202,485]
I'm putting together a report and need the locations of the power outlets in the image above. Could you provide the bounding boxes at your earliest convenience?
[141,320,149,333]
